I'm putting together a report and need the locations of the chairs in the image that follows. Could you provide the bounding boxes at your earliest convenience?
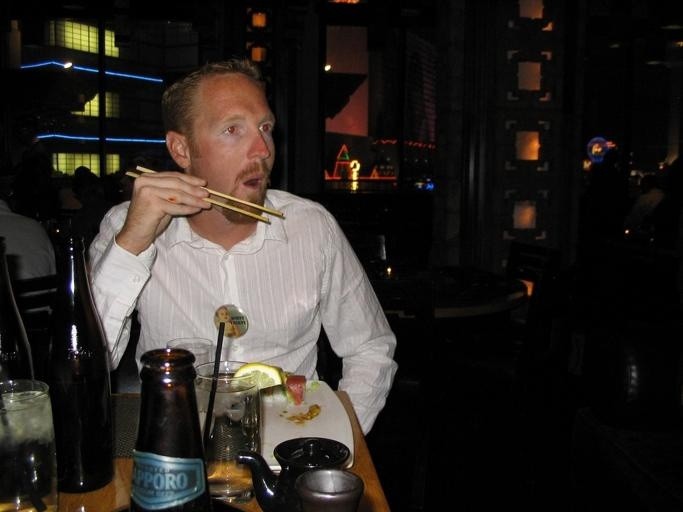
[15,272,74,336]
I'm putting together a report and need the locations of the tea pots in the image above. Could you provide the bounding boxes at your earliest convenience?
[235,436,351,512]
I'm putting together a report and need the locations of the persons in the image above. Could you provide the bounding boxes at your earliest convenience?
[645,155,683,241]
[11,117,62,224]
[86,57,399,439]
[0,151,181,314]
[625,172,668,230]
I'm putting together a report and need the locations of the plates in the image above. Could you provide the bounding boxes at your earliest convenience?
[256,379,357,470]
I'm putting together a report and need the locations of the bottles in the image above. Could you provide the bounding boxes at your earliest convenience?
[0,235,34,383]
[127,345,218,512]
[43,231,115,494]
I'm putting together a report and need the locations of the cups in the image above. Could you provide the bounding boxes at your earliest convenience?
[193,358,264,505]
[166,336,215,370]
[0,376,57,512]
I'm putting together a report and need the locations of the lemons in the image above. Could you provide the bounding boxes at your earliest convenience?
[230,361,282,393]
